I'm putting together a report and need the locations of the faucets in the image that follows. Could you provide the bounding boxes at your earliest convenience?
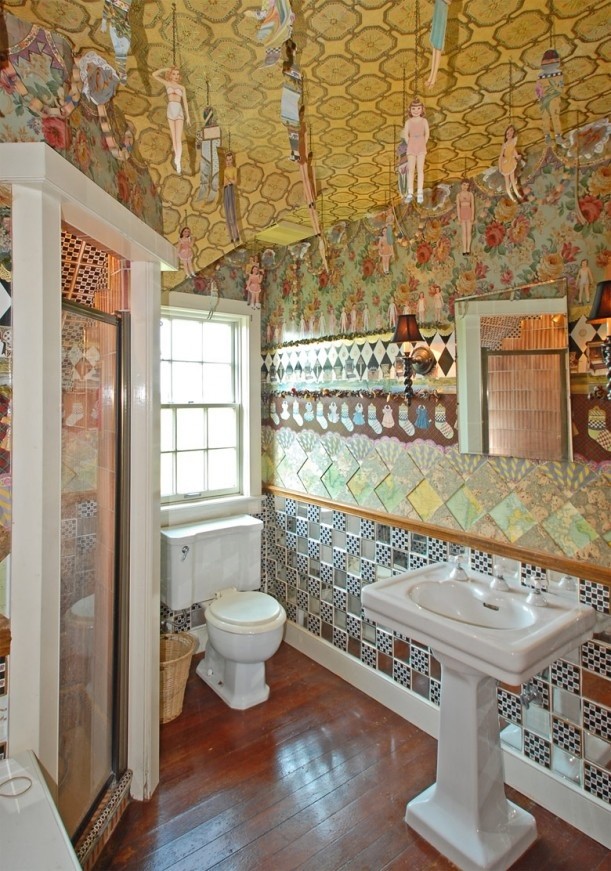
[490,564,509,592]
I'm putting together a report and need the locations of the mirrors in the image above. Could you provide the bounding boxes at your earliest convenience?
[455,277,574,462]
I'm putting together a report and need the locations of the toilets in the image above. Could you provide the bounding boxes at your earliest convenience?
[63,592,94,699]
[160,513,288,712]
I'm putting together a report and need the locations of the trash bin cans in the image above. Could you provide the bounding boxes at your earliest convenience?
[159,633,196,724]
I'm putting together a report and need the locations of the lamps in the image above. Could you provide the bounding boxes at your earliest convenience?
[586,279,611,401]
[389,313,435,407]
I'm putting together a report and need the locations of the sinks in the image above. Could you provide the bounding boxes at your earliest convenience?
[361,578,597,687]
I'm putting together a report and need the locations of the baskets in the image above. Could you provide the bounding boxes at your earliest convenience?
[159,633,198,725]
